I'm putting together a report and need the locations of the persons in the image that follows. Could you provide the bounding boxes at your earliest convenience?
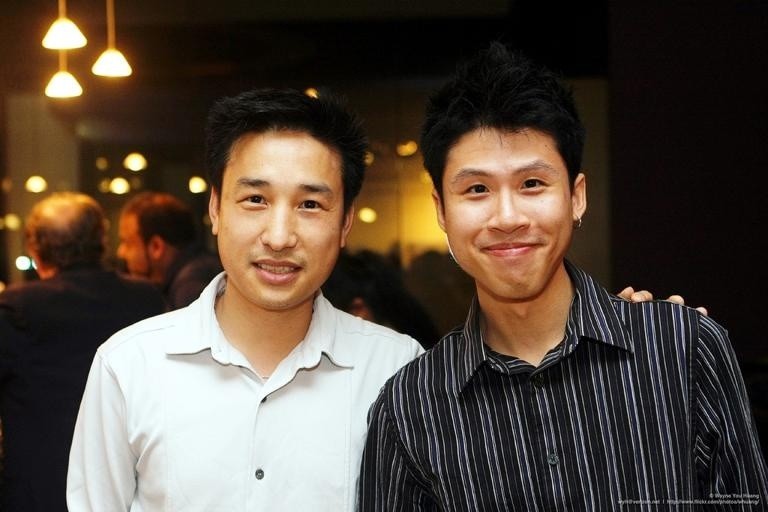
[61,88,713,512]
[351,53,765,511]
[1,190,168,512]
[318,250,442,358]
[107,190,214,312]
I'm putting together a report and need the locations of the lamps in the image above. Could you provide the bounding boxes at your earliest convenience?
[38,1,136,102]
